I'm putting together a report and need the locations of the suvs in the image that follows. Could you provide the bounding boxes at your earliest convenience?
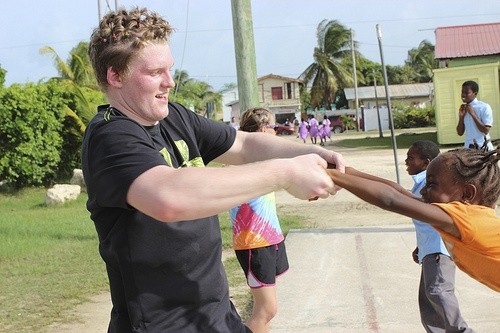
[329,113,363,134]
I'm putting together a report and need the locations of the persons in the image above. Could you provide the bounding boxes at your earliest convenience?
[79,5,343,333]
[404,141,477,333]
[308,148,500,292]
[284,111,334,147]
[229,107,290,333]
[372,100,426,109]
[229,116,240,130]
[455,80,497,155]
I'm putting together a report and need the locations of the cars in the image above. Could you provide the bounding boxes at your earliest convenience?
[274,122,294,135]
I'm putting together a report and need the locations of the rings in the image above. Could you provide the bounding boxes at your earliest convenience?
[309,196,318,201]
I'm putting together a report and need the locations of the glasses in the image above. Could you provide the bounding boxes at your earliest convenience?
[264,124,279,131]
